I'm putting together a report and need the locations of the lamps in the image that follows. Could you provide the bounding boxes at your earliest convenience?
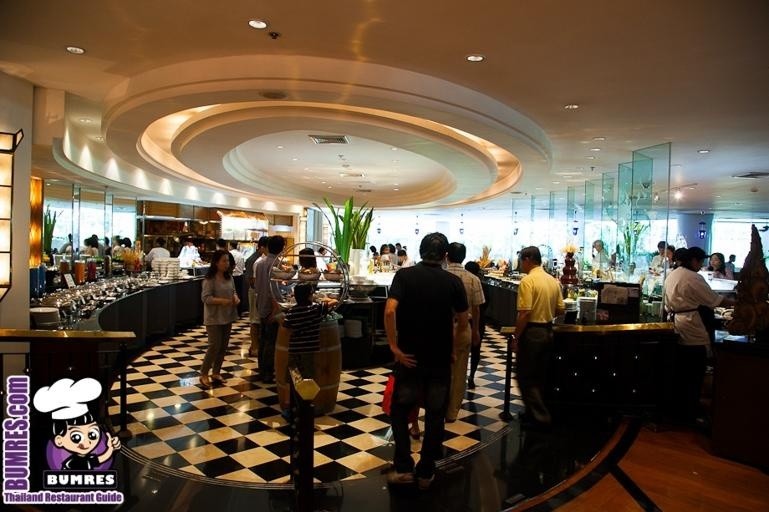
[698,218,707,240]
[0,125,25,300]
[572,210,581,235]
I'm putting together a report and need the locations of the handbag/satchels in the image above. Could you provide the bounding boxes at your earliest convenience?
[382,373,418,423]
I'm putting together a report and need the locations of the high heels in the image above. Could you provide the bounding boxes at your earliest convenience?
[211,374,227,383]
[200,378,213,390]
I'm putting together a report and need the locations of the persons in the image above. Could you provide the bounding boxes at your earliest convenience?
[442,240,486,426]
[510,244,568,433]
[670,247,717,339]
[463,260,490,393]
[660,245,740,432]
[278,283,340,422]
[227,239,245,320]
[197,248,241,390]
[381,232,471,496]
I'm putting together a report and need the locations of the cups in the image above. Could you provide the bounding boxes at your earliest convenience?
[639,292,661,318]
[381,255,390,273]
[57,258,98,288]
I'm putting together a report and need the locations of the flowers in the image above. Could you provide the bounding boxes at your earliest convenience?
[561,245,579,254]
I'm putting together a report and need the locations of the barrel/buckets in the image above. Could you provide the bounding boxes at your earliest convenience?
[273,309,343,416]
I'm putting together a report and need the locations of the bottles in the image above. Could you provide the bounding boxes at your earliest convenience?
[560,285,598,326]
[368,256,381,275]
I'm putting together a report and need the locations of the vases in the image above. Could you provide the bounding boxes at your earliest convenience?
[560,251,577,285]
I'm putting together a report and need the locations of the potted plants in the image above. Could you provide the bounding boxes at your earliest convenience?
[338,206,377,278]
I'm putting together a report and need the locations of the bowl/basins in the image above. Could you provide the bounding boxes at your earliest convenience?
[271,269,344,282]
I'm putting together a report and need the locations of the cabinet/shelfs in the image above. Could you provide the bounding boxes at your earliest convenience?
[136,198,299,269]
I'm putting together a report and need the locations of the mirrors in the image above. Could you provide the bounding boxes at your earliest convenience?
[41,174,137,269]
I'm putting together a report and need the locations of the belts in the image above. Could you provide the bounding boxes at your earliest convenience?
[528,322,553,327]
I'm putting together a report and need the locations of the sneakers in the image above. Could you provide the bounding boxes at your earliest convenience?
[409,424,421,440]
[419,473,435,491]
[380,468,416,484]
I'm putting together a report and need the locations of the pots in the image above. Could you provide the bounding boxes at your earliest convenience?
[42,273,148,321]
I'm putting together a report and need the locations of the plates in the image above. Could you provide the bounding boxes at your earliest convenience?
[151,257,181,281]
[30,306,61,329]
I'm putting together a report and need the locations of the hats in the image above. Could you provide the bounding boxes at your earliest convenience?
[31,376,103,421]
